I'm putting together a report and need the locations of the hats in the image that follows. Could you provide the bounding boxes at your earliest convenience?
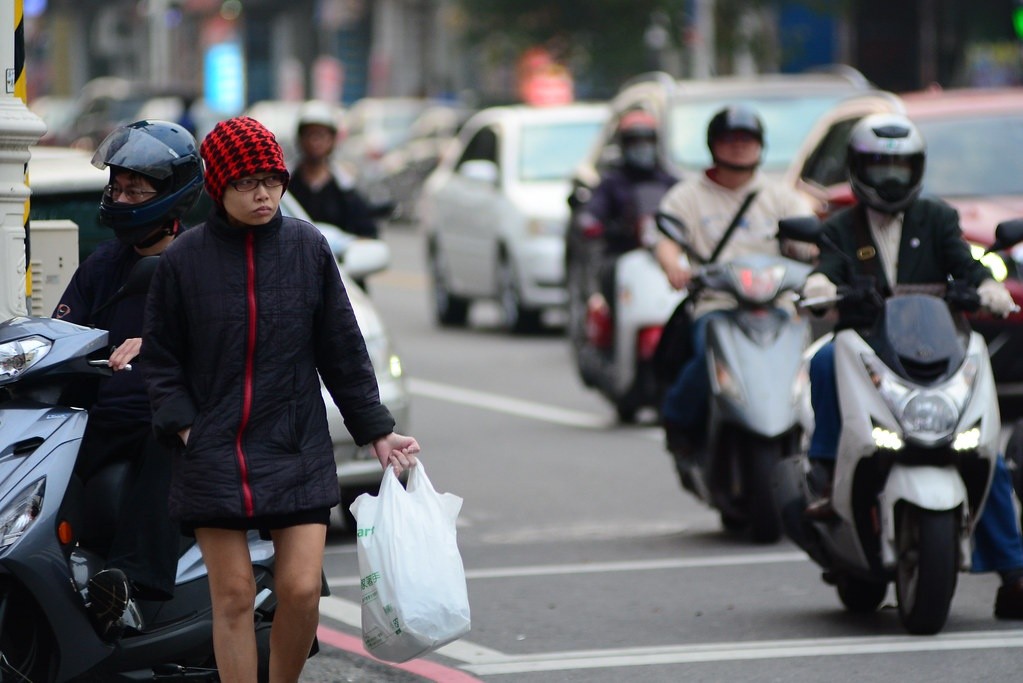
[200,117,289,205]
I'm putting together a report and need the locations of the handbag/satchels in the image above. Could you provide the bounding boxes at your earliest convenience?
[649,306,697,390]
[348,458,473,662]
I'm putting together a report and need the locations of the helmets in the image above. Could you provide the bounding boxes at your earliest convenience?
[100,118,205,247]
[619,113,659,145]
[846,112,926,213]
[707,110,764,144]
[296,101,340,132]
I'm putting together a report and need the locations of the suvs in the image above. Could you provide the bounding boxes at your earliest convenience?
[787,86,1023,400]
[565,62,874,388]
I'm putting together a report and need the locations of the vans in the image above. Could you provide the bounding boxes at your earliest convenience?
[25,146,412,534]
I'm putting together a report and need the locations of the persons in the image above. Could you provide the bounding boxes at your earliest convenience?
[646,99,822,460]
[797,110,1023,623]
[576,112,680,359]
[138,118,418,681]
[47,122,197,641]
[285,99,378,293]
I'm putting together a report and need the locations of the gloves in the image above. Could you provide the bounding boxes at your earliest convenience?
[802,273,837,298]
[980,282,1020,318]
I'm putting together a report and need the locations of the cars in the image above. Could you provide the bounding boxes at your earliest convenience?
[418,102,612,331]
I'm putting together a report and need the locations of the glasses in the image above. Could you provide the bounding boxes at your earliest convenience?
[230,174,283,193]
[104,182,157,204]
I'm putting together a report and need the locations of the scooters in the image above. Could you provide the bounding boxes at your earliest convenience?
[0,256,332,683]
[772,213,1023,636]
[569,209,817,534]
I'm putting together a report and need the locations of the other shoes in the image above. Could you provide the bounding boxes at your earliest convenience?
[808,462,833,495]
[86,568,130,626]
[994,588,1022,617]
